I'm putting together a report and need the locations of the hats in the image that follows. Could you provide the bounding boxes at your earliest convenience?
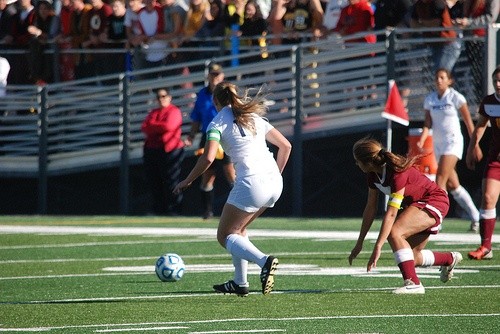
[209,61,222,74]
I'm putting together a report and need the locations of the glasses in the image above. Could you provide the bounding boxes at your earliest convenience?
[157,93,170,99]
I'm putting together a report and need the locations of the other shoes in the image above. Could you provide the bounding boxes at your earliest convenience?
[203,209,213,219]
[147,208,158,217]
[169,207,180,216]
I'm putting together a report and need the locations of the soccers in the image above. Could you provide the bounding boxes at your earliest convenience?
[155,253,185,282]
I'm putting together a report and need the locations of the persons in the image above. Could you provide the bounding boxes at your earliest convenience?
[0,0,500,156]
[140,87,184,215]
[349,138,464,294]
[172,81,293,299]
[416,68,483,233]
[466,66,500,260]
[183,64,235,220]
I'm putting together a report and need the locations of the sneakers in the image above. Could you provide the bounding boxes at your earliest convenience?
[467,246,493,260]
[393,282,425,294]
[439,251,462,283]
[213,280,249,297]
[471,221,479,232]
[260,256,279,296]
